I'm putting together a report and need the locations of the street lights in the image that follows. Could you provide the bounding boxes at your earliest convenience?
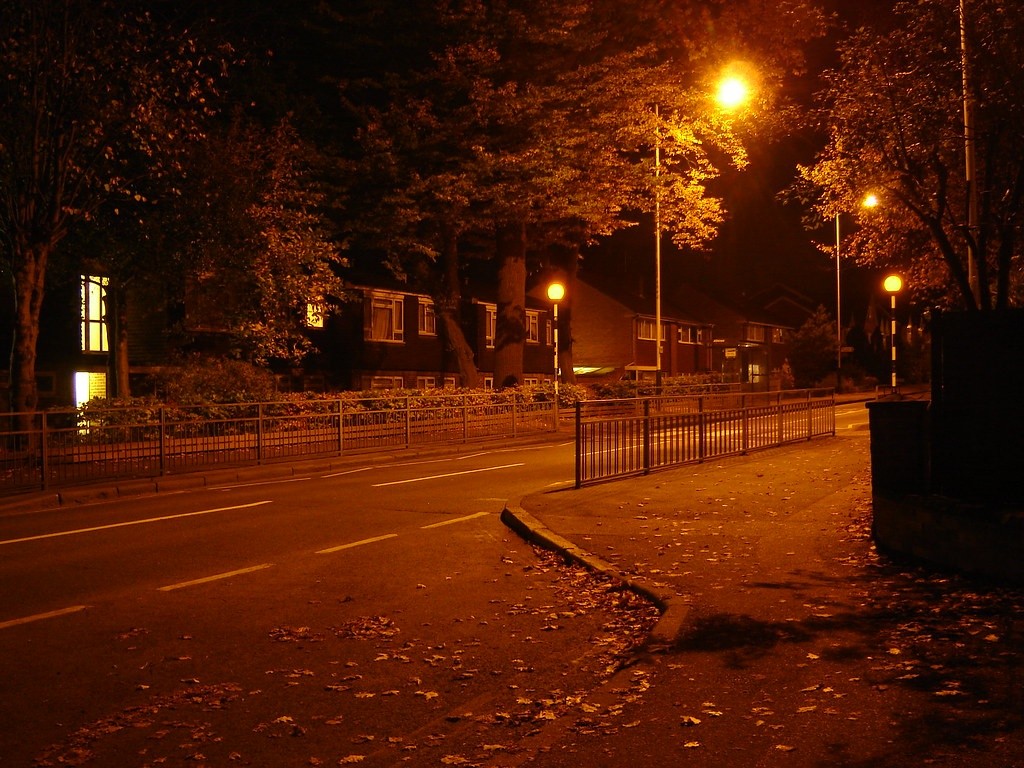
[547,282,567,430]
[835,193,878,394]
[651,67,749,403]
[884,275,901,395]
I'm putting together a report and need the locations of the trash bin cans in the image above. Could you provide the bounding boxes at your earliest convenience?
[863,394,933,556]
